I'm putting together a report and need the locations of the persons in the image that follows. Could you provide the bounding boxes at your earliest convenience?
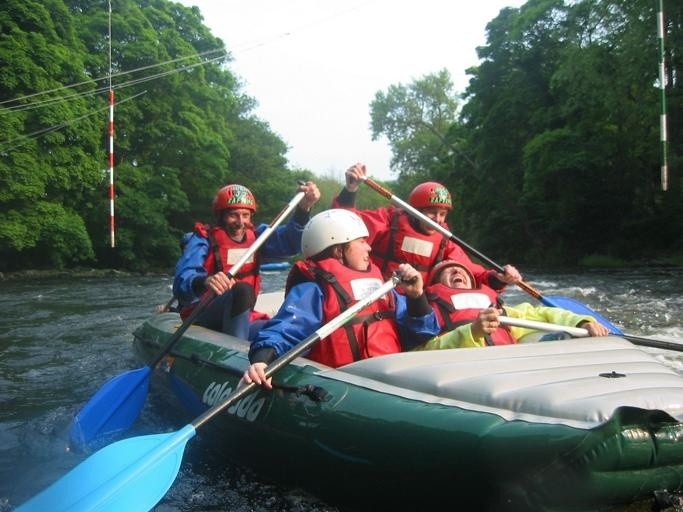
[333,162,521,295]
[155,232,194,314]
[405,259,608,352]
[172,179,321,338]
[234,209,441,399]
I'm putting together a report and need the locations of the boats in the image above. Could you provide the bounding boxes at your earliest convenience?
[256,260,291,273]
[127,287,681,511]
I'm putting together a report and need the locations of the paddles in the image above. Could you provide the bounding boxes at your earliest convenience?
[359,175,623,335]
[14,271,403,512]
[66,180,307,453]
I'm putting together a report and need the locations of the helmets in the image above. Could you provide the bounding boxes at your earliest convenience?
[409,181,452,210]
[426,259,476,290]
[212,184,257,214]
[300,208,371,262]
